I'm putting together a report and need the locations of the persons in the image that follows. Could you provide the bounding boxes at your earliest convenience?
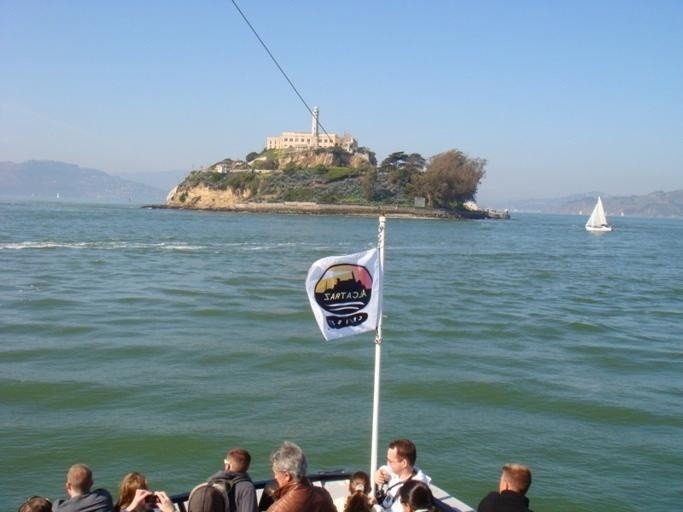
[476,463,536,511]
[17,438,444,511]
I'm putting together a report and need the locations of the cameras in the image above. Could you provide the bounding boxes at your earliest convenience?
[145,494,159,503]
[374,490,385,502]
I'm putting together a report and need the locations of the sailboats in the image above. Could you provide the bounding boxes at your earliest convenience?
[584,196,613,232]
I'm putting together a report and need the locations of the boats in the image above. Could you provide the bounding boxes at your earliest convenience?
[160,464,474,512]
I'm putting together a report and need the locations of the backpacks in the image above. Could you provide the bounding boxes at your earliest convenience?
[187,478,246,512]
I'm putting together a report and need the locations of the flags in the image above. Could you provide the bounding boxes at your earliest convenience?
[303,246,381,343]
